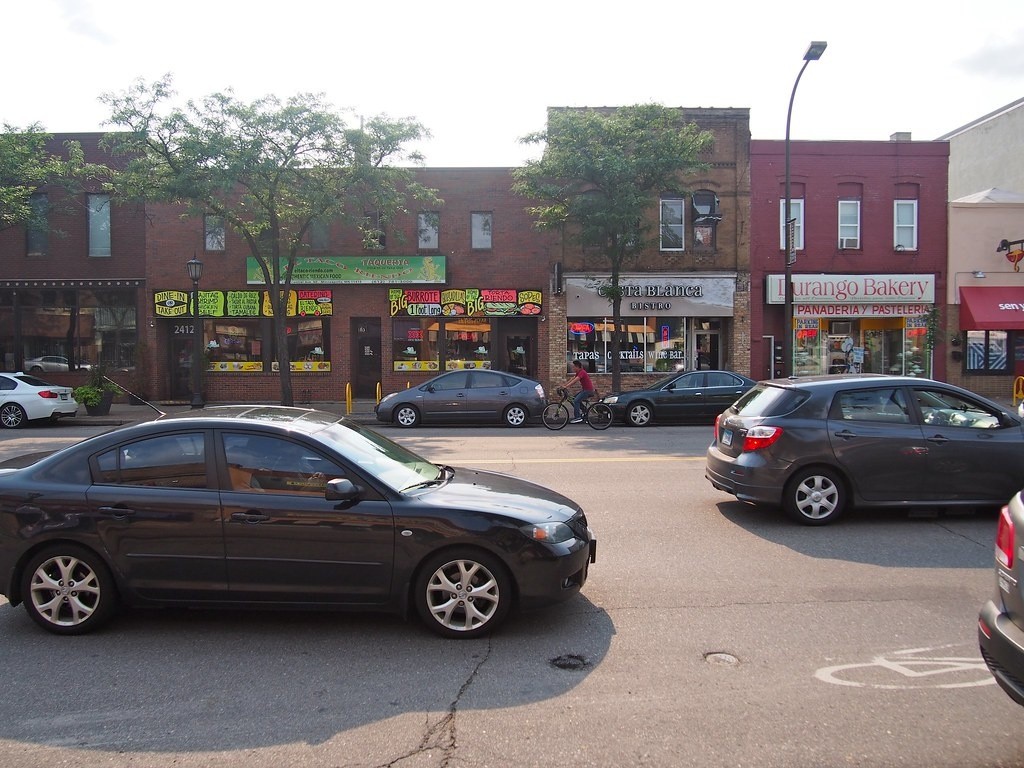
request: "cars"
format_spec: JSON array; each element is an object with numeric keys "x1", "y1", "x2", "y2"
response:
[
  {"x1": 0, "y1": 371, "x2": 79, "y2": 428},
  {"x1": 22, "y1": 356, "x2": 92, "y2": 372},
  {"x1": 374, "y1": 368, "x2": 546, "y2": 428},
  {"x1": 977, "y1": 490, "x2": 1023, "y2": 707},
  {"x1": 704, "y1": 372, "x2": 1024, "y2": 527},
  {"x1": 0, "y1": 404, "x2": 597, "y2": 639},
  {"x1": 599, "y1": 370, "x2": 757, "y2": 427}
]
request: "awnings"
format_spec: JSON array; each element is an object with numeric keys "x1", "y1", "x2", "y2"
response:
[
  {"x1": 567, "y1": 322, "x2": 655, "y2": 333},
  {"x1": 0, "y1": 314, "x2": 95, "y2": 342},
  {"x1": 959, "y1": 285, "x2": 1024, "y2": 331},
  {"x1": 427, "y1": 322, "x2": 490, "y2": 332}
]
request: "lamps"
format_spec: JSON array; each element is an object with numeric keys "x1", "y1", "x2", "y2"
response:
[{"x1": 972, "y1": 271, "x2": 986, "y2": 278}]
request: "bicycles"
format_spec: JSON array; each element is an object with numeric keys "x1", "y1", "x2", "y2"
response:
[{"x1": 542, "y1": 388, "x2": 613, "y2": 431}]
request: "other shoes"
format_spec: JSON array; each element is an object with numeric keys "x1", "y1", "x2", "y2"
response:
[{"x1": 570, "y1": 417, "x2": 582, "y2": 423}]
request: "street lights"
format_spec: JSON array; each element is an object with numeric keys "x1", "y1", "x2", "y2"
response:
[
  {"x1": 784, "y1": 40, "x2": 829, "y2": 374},
  {"x1": 186, "y1": 249, "x2": 205, "y2": 410}
]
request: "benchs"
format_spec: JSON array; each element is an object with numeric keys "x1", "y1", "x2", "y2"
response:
[{"x1": 97, "y1": 445, "x2": 147, "y2": 485}]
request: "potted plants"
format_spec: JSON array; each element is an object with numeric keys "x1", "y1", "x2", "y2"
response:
[{"x1": 73, "y1": 361, "x2": 123, "y2": 416}]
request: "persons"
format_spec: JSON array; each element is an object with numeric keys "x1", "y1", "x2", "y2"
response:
[
  {"x1": 269, "y1": 436, "x2": 327, "y2": 491},
  {"x1": 556, "y1": 360, "x2": 595, "y2": 424}
]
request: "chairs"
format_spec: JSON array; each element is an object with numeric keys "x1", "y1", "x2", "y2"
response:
[
  {"x1": 227, "y1": 445, "x2": 263, "y2": 493},
  {"x1": 246, "y1": 439, "x2": 268, "y2": 469}
]
